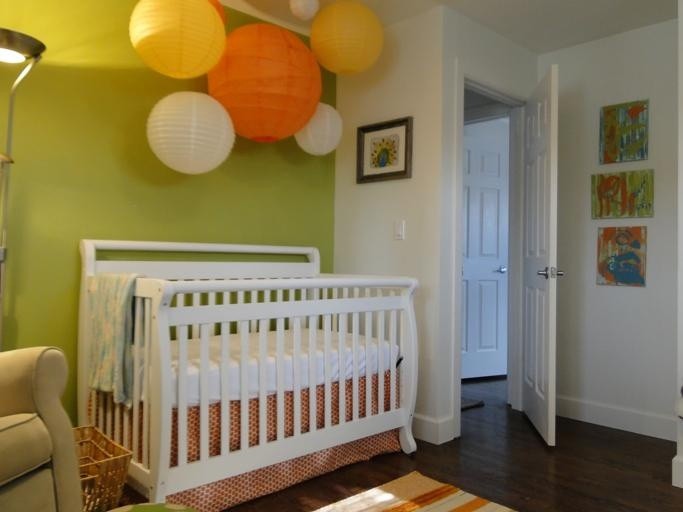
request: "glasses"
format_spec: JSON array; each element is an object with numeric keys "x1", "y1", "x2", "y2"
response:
[
  {"x1": 289, "y1": 0, "x2": 320, "y2": 21},
  {"x1": 294, "y1": 103, "x2": 344, "y2": 156},
  {"x1": 144, "y1": 91, "x2": 236, "y2": 176},
  {"x1": 209, "y1": 1, "x2": 228, "y2": 27},
  {"x1": 310, "y1": 0, "x2": 384, "y2": 76},
  {"x1": 0, "y1": 27, "x2": 47, "y2": 325},
  {"x1": 206, "y1": 22, "x2": 322, "y2": 143},
  {"x1": 128, "y1": 1, "x2": 227, "y2": 79}
]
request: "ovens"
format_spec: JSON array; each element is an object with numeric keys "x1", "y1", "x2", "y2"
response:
[{"x1": 75, "y1": 239, "x2": 418, "y2": 512}]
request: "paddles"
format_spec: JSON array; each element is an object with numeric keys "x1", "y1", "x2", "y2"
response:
[
  {"x1": 312, "y1": 469, "x2": 518, "y2": 512},
  {"x1": 461, "y1": 396, "x2": 485, "y2": 411}
]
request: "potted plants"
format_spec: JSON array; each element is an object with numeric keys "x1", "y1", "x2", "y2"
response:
[{"x1": 355, "y1": 116, "x2": 413, "y2": 184}]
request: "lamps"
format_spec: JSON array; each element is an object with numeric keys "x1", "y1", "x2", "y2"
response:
[{"x1": 0, "y1": 346, "x2": 84, "y2": 512}]
